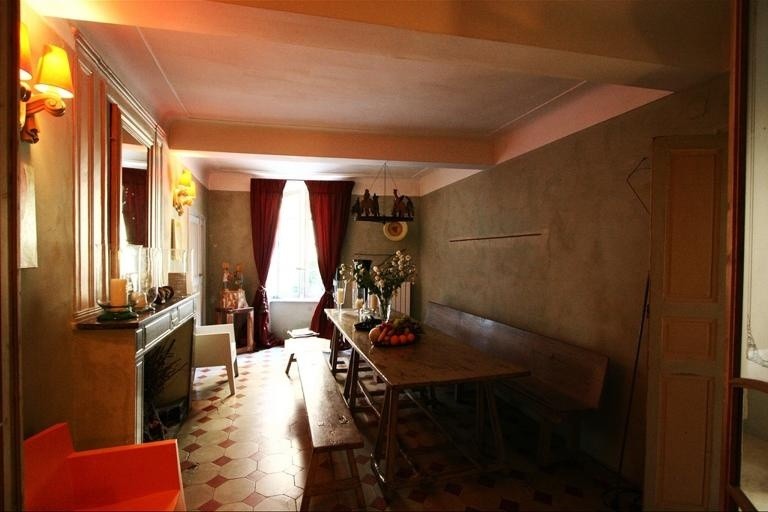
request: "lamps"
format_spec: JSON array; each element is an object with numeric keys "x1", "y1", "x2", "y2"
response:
[
  {"x1": 168, "y1": 160, "x2": 197, "y2": 216},
  {"x1": 18, "y1": 23, "x2": 75, "y2": 144}
]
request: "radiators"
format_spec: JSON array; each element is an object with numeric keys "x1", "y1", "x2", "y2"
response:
[{"x1": 351, "y1": 282, "x2": 411, "y2": 317}]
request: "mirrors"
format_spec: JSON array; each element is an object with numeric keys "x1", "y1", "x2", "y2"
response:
[{"x1": 111, "y1": 109, "x2": 161, "y2": 296}]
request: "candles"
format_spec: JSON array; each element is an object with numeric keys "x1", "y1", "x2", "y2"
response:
[{"x1": 109, "y1": 278, "x2": 129, "y2": 308}]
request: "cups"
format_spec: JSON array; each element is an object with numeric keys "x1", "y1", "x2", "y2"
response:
[{"x1": 120, "y1": 244, "x2": 188, "y2": 312}]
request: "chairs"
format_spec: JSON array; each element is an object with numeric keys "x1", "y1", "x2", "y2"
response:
[
  {"x1": 188, "y1": 318, "x2": 240, "y2": 398},
  {"x1": 20, "y1": 422, "x2": 188, "y2": 512}
]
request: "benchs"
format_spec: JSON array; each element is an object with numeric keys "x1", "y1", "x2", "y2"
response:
[
  {"x1": 284, "y1": 346, "x2": 367, "y2": 511},
  {"x1": 423, "y1": 300, "x2": 610, "y2": 472}
]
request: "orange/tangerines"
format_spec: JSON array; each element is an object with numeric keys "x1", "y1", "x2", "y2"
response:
[
  {"x1": 390, "y1": 335, "x2": 399, "y2": 344},
  {"x1": 407, "y1": 333, "x2": 415, "y2": 341},
  {"x1": 399, "y1": 334, "x2": 406, "y2": 344}
]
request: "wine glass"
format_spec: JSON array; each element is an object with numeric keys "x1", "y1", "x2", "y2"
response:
[{"x1": 333, "y1": 280, "x2": 379, "y2": 318}]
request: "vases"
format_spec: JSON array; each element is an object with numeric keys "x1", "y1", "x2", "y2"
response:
[{"x1": 379, "y1": 301, "x2": 392, "y2": 325}]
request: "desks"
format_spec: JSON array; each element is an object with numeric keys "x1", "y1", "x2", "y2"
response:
[{"x1": 322, "y1": 304, "x2": 532, "y2": 501}]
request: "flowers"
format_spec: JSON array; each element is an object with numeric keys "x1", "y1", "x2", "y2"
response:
[{"x1": 339, "y1": 250, "x2": 418, "y2": 322}]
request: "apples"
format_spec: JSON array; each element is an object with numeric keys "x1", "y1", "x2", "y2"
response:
[{"x1": 369, "y1": 327, "x2": 380, "y2": 342}]
